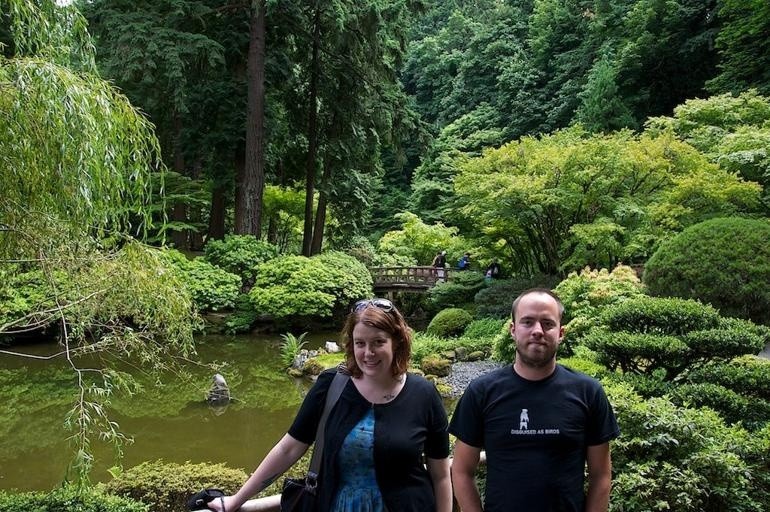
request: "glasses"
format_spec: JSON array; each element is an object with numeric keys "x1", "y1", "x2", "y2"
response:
[{"x1": 352, "y1": 299, "x2": 393, "y2": 312}]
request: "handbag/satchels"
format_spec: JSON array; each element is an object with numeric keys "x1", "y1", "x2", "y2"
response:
[{"x1": 280, "y1": 477, "x2": 317, "y2": 512}]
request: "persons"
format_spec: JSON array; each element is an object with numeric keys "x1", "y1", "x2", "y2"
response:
[
  {"x1": 206, "y1": 296, "x2": 454, "y2": 512},
  {"x1": 448, "y1": 286, "x2": 620, "y2": 512},
  {"x1": 432, "y1": 252, "x2": 446, "y2": 269},
  {"x1": 459, "y1": 252, "x2": 472, "y2": 271},
  {"x1": 487, "y1": 257, "x2": 501, "y2": 280}
]
